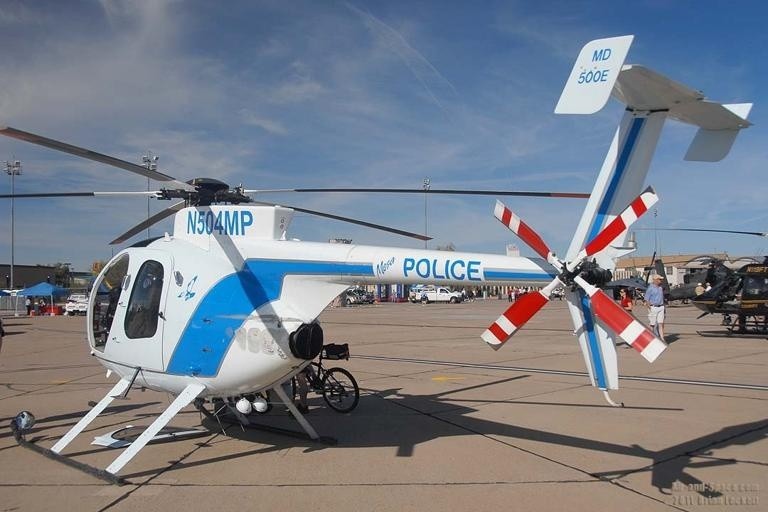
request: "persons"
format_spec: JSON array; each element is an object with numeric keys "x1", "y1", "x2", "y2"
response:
[
  {"x1": 24, "y1": 296, "x2": 47, "y2": 317},
  {"x1": 354, "y1": 285, "x2": 566, "y2": 304},
  {"x1": 288, "y1": 367, "x2": 309, "y2": 417},
  {"x1": 616, "y1": 288, "x2": 635, "y2": 349},
  {"x1": 643, "y1": 273, "x2": 667, "y2": 343},
  {"x1": 632, "y1": 281, "x2": 712, "y2": 305}
]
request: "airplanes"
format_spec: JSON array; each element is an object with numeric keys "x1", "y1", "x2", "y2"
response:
[{"x1": 600, "y1": 259, "x2": 706, "y2": 302}]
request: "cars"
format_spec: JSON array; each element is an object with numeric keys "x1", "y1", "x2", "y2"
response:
[{"x1": 347, "y1": 289, "x2": 375, "y2": 305}]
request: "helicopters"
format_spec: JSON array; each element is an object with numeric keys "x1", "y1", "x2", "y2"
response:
[
  {"x1": 0, "y1": 35, "x2": 753, "y2": 487},
  {"x1": 693, "y1": 256, "x2": 767, "y2": 339}
]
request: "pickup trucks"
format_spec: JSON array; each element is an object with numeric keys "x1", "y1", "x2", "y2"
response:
[
  {"x1": 416, "y1": 288, "x2": 464, "y2": 303},
  {"x1": 66, "y1": 294, "x2": 88, "y2": 315}
]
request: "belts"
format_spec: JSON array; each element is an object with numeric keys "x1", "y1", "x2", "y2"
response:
[{"x1": 649, "y1": 304, "x2": 664, "y2": 307}]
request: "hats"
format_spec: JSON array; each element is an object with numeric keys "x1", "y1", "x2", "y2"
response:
[
  {"x1": 697, "y1": 282, "x2": 703, "y2": 286},
  {"x1": 706, "y1": 283, "x2": 711, "y2": 286},
  {"x1": 652, "y1": 274, "x2": 665, "y2": 281}
]
